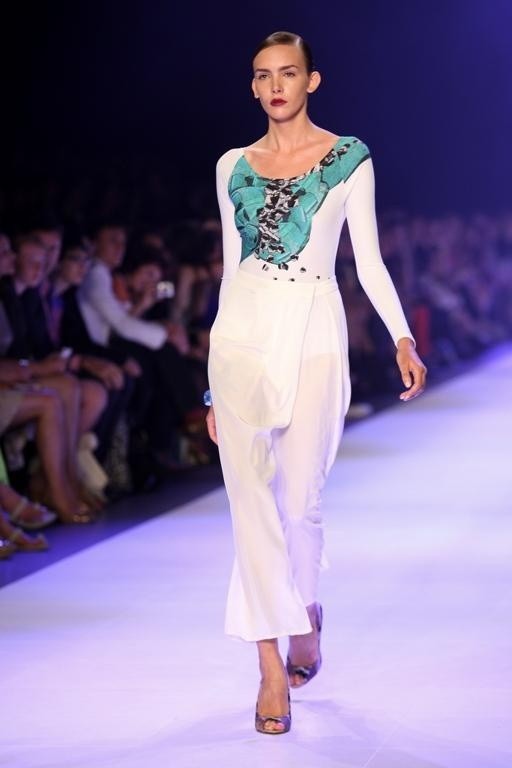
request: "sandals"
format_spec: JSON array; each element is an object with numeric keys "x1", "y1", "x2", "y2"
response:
[
  {"x1": 4, "y1": 531, "x2": 49, "y2": 551},
  {"x1": 6, "y1": 499, "x2": 56, "y2": 528}
]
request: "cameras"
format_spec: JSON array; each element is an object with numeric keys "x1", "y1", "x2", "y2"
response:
[{"x1": 153, "y1": 281, "x2": 175, "y2": 300}]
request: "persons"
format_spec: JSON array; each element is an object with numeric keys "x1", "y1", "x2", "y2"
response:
[
  {"x1": 206, "y1": 30, "x2": 429, "y2": 735},
  {"x1": 335, "y1": 212, "x2": 512, "y2": 425},
  {"x1": 2, "y1": 214, "x2": 225, "y2": 562}
]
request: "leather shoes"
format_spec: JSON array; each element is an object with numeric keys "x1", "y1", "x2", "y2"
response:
[
  {"x1": 255, "y1": 672, "x2": 291, "y2": 734},
  {"x1": 286, "y1": 607, "x2": 323, "y2": 687}
]
